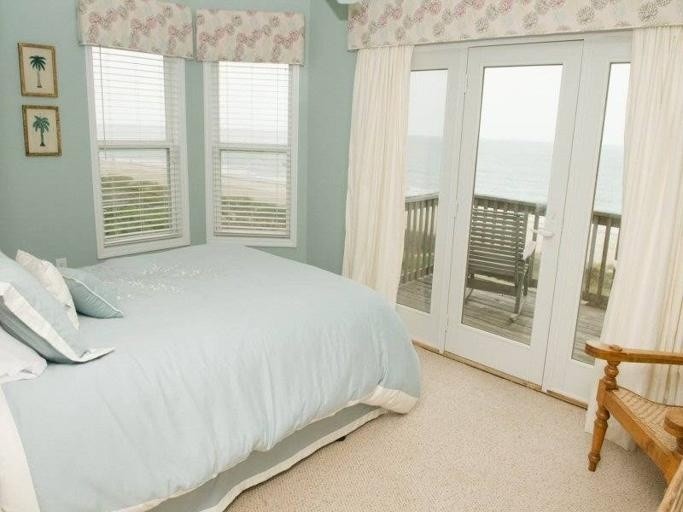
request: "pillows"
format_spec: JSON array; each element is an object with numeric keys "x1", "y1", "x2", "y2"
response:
[
  {"x1": 17, "y1": 249, "x2": 79, "y2": 329},
  {"x1": 56, "y1": 267, "x2": 123, "y2": 318},
  {"x1": 0, "y1": 249, "x2": 114, "y2": 364}
]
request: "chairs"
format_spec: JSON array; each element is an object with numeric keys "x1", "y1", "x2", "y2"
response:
[
  {"x1": 464, "y1": 206, "x2": 536, "y2": 321},
  {"x1": 583, "y1": 339, "x2": 683, "y2": 487}
]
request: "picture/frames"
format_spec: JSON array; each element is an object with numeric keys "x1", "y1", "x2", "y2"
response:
[
  {"x1": 22, "y1": 105, "x2": 63, "y2": 157},
  {"x1": 47, "y1": 75, "x2": 54, "y2": 76},
  {"x1": 18, "y1": 43, "x2": 58, "y2": 97}
]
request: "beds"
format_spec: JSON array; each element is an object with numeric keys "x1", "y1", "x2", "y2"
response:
[{"x1": 0, "y1": 244, "x2": 421, "y2": 512}]
